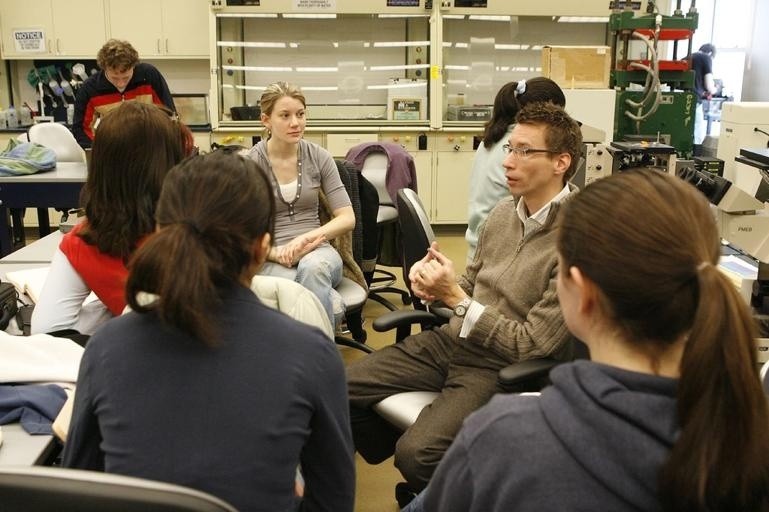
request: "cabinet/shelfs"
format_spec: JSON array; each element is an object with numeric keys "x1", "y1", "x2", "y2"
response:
[
  {"x1": 0, "y1": 1, "x2": 110, "y2": 65},
  {"x1": 205, "y1": 0, "x2": 619, "y2": 228},
  {"x1": 102, "y1": 1, "x2": 212, "y2": 64}
]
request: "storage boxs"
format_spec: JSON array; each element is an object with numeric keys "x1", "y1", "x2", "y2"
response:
[{"x1": 540, "y1": 44, "x2": 613, "y2": 91}]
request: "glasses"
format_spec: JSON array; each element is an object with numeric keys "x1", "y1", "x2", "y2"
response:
[{"x1": 502, "y1": 144, "x2": 563, "y2": 158}]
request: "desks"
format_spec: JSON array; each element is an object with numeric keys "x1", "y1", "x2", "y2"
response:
[
  {"x1": 0, "y1": 161, "x2": 89, "y2": 263},
  {"x1": 0, "y1": 229, "x2": 66, "y2": 263},
  {"x1": 0, "y1": 261, "x2": 103, "y2": 470}
]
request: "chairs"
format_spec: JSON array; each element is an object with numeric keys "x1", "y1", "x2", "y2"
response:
[
  {"x1": 0, "y1": 462, "x2": 239, "y2": 511},
  {"x1": 311, "y1": 136, "x2": 462, "y2": 361},
  {"x1": 365, "y1": 302, "x2": 568, "y2": 511}
]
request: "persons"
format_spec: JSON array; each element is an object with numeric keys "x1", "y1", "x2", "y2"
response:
[
  {"x1": 466, "y1": 77, "x2": 566, "y2": 271},
  {"x1": 73, "y1": 39, "x2": 177, "y2": 150},
  {"x1": 30, "y1": 102, "x2": 185, "y2": 339},
  {"x1": 344, "y1": 102, "x2": 583, "y2": 496},
  {"x1": 680, "y1": 44, "x2": 718, "y2": 144},
  {"x1": 242, "y1": 83, "x2": 357, "y2": 338},
  {"x1": 60, "y1": 149, "x2": 357, "y2": 512},
  {"x1": 398, "y1": 168, "x2": 769, "y2": 512}
]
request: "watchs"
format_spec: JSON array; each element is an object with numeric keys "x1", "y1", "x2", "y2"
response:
[{"x1": 453, "y1": 298, "x2": 471, "y2": 317}]
